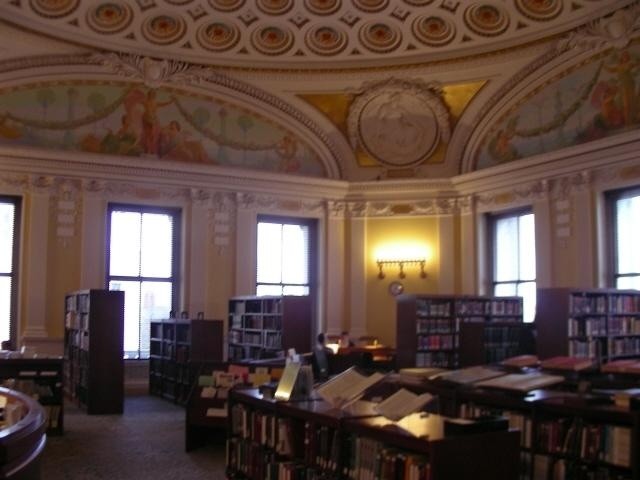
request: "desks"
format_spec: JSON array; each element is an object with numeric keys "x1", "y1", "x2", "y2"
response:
[{"x1": 1, "y1": 339, "x2": 67, "y2": 437}]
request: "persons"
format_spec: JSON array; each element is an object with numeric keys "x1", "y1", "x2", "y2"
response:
[
  {"x1": 337, "y1": 332, "x2": 354, "y2": 348},
  {"x1": 318, "y1": 333, "x2": 333, "y2": 359}
]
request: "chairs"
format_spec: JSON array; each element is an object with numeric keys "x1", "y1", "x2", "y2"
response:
[{"x1": 313, "y1": 347, "x2": 378, "y2": 382}]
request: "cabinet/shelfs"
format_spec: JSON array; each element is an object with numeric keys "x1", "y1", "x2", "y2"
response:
[
  {"x1": 368, "y1": 354, "x2": 640, "y2": 479},
  {"x1": 184, "y1": 359, "x2": 313, "y2": 449},
  {"x1": 393, "y1": 283, "x2": 640, "y2": 368},
  {"x1": 227, "y1": 383, "x2": 522, "y2": 478},
  {"x1": 61, "y1": 287, "x2": 313, "y2": 416}
]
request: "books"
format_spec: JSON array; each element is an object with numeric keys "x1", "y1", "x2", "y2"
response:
[
  {"x1": 228, "y1": 300, "x2": 282, "y2": 362},
  {"x1": 61, "y1": 295, "x2": 90, "y2": 402},
  {"x1": 415, "y1": 298, "x2": 522, "y2": 368},
  {"x1": 2, "y1": 347, "x2": 64, "y2": 429},
  {"x1": 199, "y1": 350, "x2": 640, "y2": 480},
  {"x1": 567, "y1": 296, "x2": 639, "y2": 361}
]
